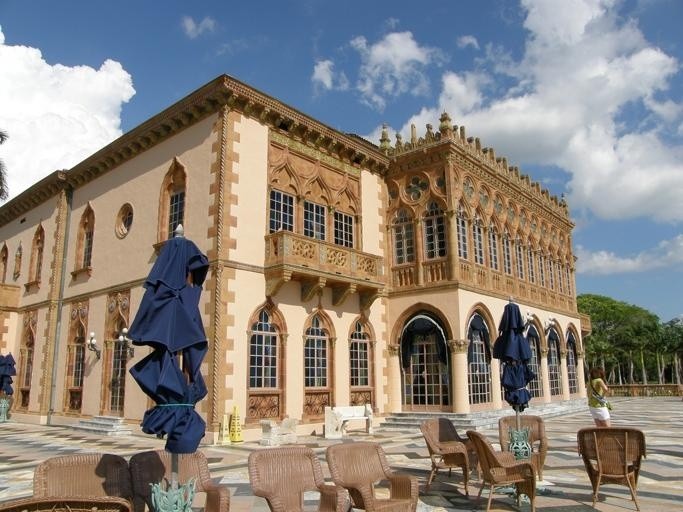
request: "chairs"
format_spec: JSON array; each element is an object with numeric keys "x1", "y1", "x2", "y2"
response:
[
  {"x1": 34, "y1": 453, "x2": 134, "y2": 512},
  {"x1": 577, "y1": 427, "x2": 646, "y2": 512},
  {"x1": 420, "y1": 418, "x2": 481, "y2": 497},
  {"x1": 499, "y1": 416, "x2": 547, "y2": 482},
  {"x1": 0, "y1": 496, "x2": 134, "y2": 512},
  {"x1": 249, "y1": 445, "x2": 345, "y2": 512},
  {"x1": 327, "y1": 443, "x2": 419, "y2": 512},
  {"x1": 466, "y1": 431, "x2": 536, "y2": 512},
  {"x1": 130, "y1": 449, "x2": 230, "y2": 512}
]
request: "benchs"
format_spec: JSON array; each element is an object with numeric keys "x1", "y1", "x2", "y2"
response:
[{"x1": 324, "y1": 404, "x2": 373, "y2": 439}]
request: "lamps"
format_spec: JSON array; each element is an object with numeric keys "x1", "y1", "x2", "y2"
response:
[
  {"x1": 86, "y1": 331, "x2": 100, "y2": 359},
  {"x1": 118, "y1": 328, "x2": 134, "y2": 357}
]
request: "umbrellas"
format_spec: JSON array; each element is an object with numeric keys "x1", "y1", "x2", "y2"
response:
[
  {"x1": 493, "y1": 295, "x2": 535, "y2": 430},
  {"x1": 125, "y1": 223, "x2": 210, "y2": 488},
  {"x1": 0, "y1": 352, "x2": 16, "y2": 399}
]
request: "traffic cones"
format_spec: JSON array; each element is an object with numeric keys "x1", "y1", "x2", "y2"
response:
[{"x1": 230, "y1": 405, "x2": 243, "y2": 442}]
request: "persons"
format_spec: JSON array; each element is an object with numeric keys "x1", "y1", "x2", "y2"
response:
[{"x1": 587, "y1": 366, "x2": 612, "y2": 427}]
